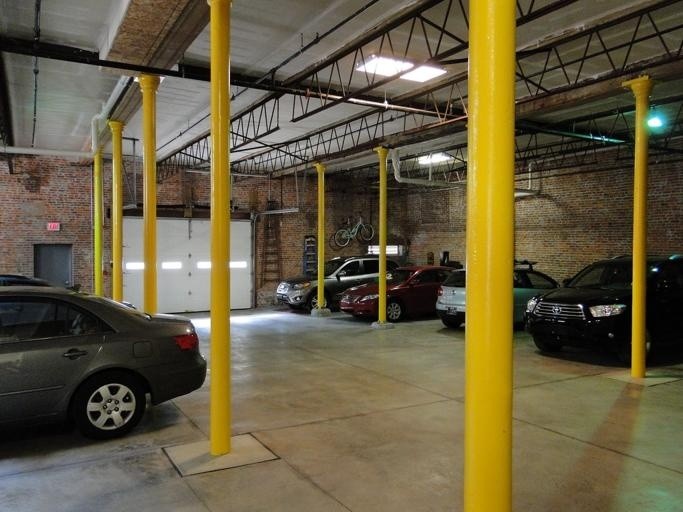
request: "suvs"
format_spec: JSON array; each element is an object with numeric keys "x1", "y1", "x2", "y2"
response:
[
  {"x1": 275, "y1": 253, "x2": 401, "y2": 312},
  {"x1": 524, "y1": 254, "x2": 683, "y2": 361}
]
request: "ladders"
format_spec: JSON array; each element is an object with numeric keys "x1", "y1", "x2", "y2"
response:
[
  {"x1": 304, "y1": 235, "x2": 318, "y2": 274},
  {"x1": 260, "y1": 201, "x2": 282, "y2": 288}
]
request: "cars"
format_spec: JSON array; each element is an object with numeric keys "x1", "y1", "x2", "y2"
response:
[
  {"x1": 0, "y1": 284, "x2": 206, "y2": 440},
  {"x1": 436, "y1": 263, "x2": 560, "y2": 327},
  {"x1": 338, "y1": 262, "x2": 454, "y2": 322}
]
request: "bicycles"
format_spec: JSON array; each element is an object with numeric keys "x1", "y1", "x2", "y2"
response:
[{"x1": 333, "y1": 214, "x2": 376, "y2": 248}]
